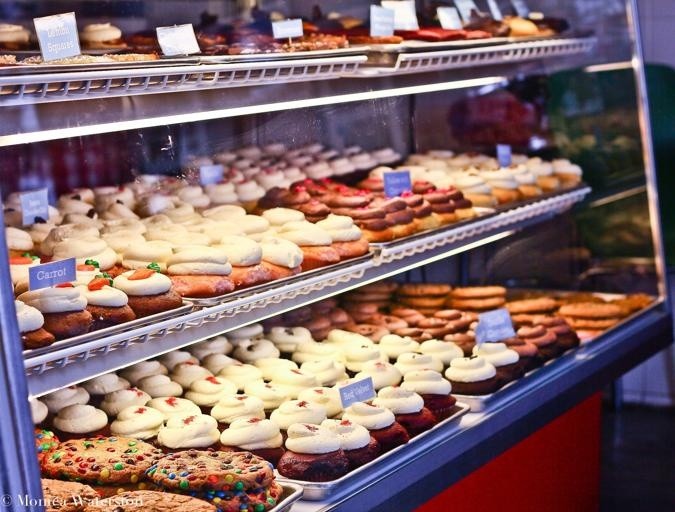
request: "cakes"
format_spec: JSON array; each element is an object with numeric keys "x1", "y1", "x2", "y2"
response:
[{"x1": 0, "y1": 11, "x2": 659, "y2": 512}]
